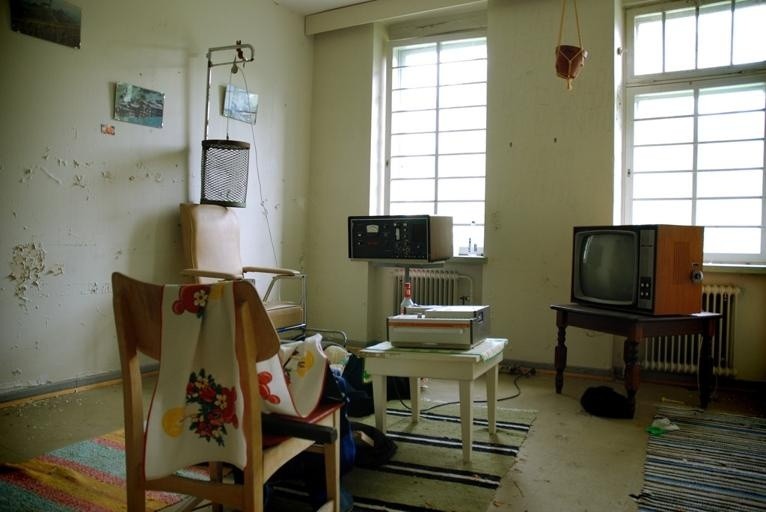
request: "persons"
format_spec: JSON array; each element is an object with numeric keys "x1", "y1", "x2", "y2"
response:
[{"x1": 118, "y1": 87, "x2": 163, "y2": 111}]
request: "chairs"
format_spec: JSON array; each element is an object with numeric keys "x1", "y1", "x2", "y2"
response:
[
  {"x1": 176, "y1": 201, "x2": 307, "y2": 336},
  {"x1": 110, "y1": 269, "x2": 341, "y2": 512}
]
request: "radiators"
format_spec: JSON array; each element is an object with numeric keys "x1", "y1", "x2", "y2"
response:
[
  {"x1": 392, "y1": 269, "x2": 474, "y2": 317},
  {"x1": 638, "y1": 285, "x2": 743, "y2": 377}
]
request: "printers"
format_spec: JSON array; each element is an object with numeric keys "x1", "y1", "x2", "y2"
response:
[{"x1": 388, "y1": 304, "x2": 490, "y2": 349}]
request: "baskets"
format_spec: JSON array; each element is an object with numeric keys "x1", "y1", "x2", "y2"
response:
[{"x1": 198, "y1": 139, "x2": 251, "y2": 208}]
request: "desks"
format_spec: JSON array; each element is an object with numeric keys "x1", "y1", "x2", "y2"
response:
[
  {"x1": 357, "y1": 336, "x2": 510, "y2": 465},
  {"x1": 550, "y1": 304, "x2": 724, "y2": 418}
]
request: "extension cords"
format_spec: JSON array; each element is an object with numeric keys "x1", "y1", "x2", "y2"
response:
[{"x1": 499, "y1": 364, "x2": 536, "y2": 375}]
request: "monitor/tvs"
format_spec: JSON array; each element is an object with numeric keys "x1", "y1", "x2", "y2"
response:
[{"x1": 571, "y1": 226, "x2": 703, "y2": 316}]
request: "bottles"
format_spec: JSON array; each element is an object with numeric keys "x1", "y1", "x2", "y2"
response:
[{"x1": 400, "y1": 283, "x2": 414, "y2": 314}]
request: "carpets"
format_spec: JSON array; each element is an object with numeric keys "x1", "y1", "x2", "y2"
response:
[{"x1": 636, "y1": 397, "x2": 766, "y2": 511}]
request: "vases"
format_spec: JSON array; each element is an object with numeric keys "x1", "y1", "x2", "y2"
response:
[{"x1": 556, "y1": 44, "x2": 588, "y2": 81}]
